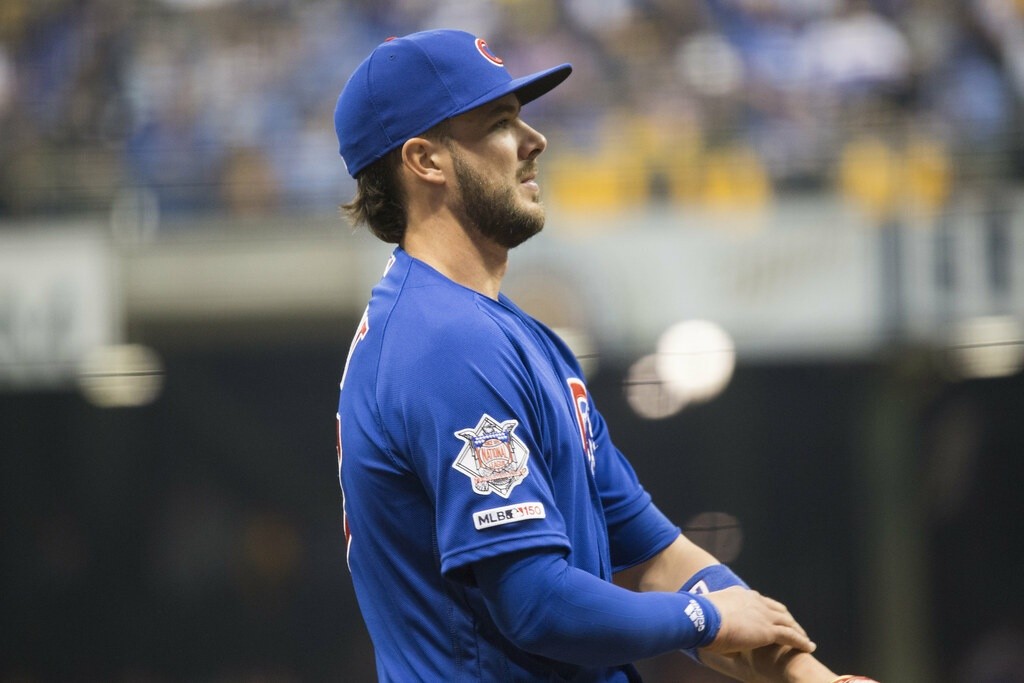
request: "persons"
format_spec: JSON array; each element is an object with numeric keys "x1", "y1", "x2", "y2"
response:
[{"x1": 327, "y1": 26, "x2": 851, "y2": 682}]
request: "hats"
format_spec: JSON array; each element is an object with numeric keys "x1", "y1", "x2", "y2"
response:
[{"x1": 334, "y1": 29, "x2": 572, "y2": 179}]
